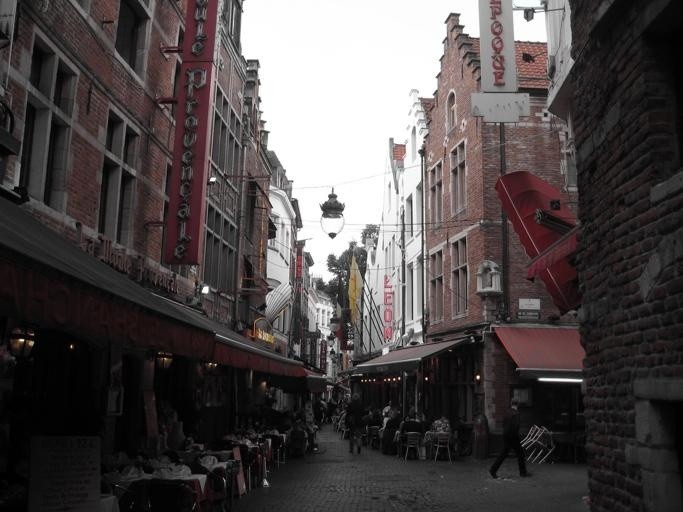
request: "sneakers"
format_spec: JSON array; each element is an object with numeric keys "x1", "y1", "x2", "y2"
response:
[{"x1": 488, "y1": 468, "x2": 532, "y2": 480}]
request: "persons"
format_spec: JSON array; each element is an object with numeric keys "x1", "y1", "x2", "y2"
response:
[
  {"x1": 341, "y1": 391, "x2": 452, "y2": 460},
  {"x1": 158, "y1": 398, "x2": 337, "y2": 490},
  {"x1": 488, "y1": 396, "x2": 532, "y2": 479}
]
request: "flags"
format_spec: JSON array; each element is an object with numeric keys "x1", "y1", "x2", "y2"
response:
[{"x1": 347, "y1": 255, "x2": 364, "y2": 325}]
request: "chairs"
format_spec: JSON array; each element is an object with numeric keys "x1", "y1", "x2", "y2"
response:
[
  {"x1": 331, "y1": 408, "x2": 452, "y2": 465},
  {"x1": 520, "y1": 423, "x2": 556, "y2": 465},
  {"x1": 102, "y1": 427, "x2": 289, "y2": 512}
]
